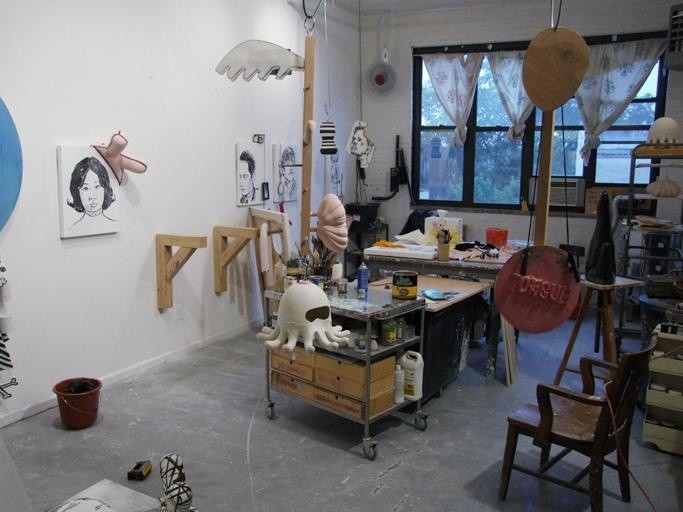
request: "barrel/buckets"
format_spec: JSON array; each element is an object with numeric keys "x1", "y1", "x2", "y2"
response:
[
  {"x1": 345, "y1": 202, "x2": 378, "y2": 232},
  {"x1": 396, "y1": 350, "x2": 424, "y2": 402}
]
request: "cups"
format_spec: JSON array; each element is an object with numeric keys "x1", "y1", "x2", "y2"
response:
[
  {"x1": 284, "y1": 259, "x2": 332, "y2": 291},
  {"x1": 337, "y1": 277, "x2": 348, "y2": 295},
  {"x1": 437, "y1": 243, "x2": 450, "y2": 262}
]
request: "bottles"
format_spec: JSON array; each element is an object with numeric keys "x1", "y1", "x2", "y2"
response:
[
  {"x1": 379, "y1": 318, "x2": 414, "y2": 346},
  {"x1": 357, "y1": 262, "x2": 369, "y2": 301}
]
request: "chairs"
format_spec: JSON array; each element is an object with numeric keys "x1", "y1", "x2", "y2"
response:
[{"x1": 498, "y1": 332, "x2": 659, "y2": 511}]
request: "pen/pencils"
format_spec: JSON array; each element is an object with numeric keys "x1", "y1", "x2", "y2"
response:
[
  {"x1": 450, "y1": 275, "x2": 481, "y2": 282},
  {"x1": 437, "y1": 228, "x2": 452, "y2": 244}
]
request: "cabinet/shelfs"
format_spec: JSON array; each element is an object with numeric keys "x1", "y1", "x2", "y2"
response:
[{"x1": 616, "y1": 142, "x2": 683, "y2": 456}]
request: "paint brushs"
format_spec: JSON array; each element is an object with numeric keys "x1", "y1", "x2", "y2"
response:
[{"x1": 295, "y1": 240, "x2": 338, "y2": 272}]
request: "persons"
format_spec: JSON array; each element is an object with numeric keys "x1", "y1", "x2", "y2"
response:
[
  {"x1": 237, "y1": 151, "x2": 260, "y2": 206},
  {"x1": 277, "y1": 147, "x2": 297, "y2": 203},
  {"x1": 349, "y1": 126, "x2": 375, "y2": 170},
  {"x1": 61, "y1": 157, "x2": 117, "y2": 238}
]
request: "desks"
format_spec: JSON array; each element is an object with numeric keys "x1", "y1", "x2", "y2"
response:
[
  {"x1": 343, "y1": 224, "x2": 388, "y2": 280},
  {"x1": 554, "y1": 273, "x2": 646, "y2": 386},
  {"x1": 370, "y1": 274, "x2": 492, "y2": 407},
  {"x1": 368, "y1": 255, "x2": 504, "y2": 385}
]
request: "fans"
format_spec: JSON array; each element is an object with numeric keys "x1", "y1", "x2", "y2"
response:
[{"x1": 366, "y1": 61, "x2": 398, "y2": 96}]
